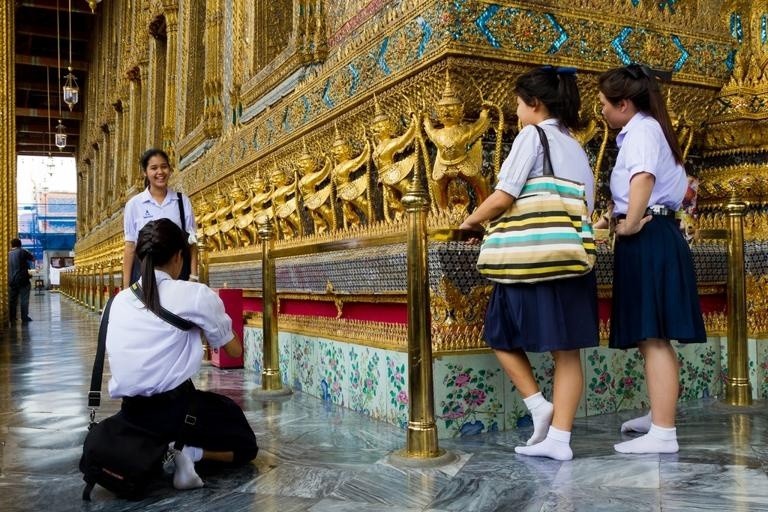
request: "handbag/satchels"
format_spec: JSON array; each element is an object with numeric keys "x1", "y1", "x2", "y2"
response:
[
  {"x1": 79, "y1": 409, "x2": 173, "y2": 502},
  {"x1": 476, "y1": 176, "x2": 597, "y2": 287},
  {"x1": 182, "y1": 233, "x2": 193, "y2": 283}
]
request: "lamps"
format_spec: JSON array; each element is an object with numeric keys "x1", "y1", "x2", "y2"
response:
[{"x1": 46, "y1": 0, "x2": 101, "y2": 176}]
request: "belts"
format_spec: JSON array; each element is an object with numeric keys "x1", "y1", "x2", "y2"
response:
[{"x1": 617, "y1": 206, "x2": 677, "y2": 218}]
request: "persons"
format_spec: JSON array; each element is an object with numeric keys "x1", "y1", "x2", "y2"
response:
[
  {"x1": 8, "y1": 320, "x2": 34, "y2": 399},
  {"x1": 201, "y1": 160, "x2": 276, "y2": 253},
  {"x1": 371, "y1": 93, "x2": 416, "y2": 224},
  {"x1": 454, "y1": 65, "x2": 599, "y2": 463},
  {"x1": 271, "y1": 137, "x2": 333, "y2": 235},
  {"x1": 424, "y1": 97, "x2": 490, "y2": 217},
  {"x1": 592, "y1": 64, "x2": 707, "y2": 455},
  {"x1": 331, "y1": 120, "x2": 375, "y2": 225},
  {"x1": 99, "y1": 217, "x2": 260, "y2": 491},
  {"x1": 7, "y1": 237, "x2": 38, "y2": 323},
  {"x1": 119, "y1": 147, "x2": 200, "y2": 290}
]
